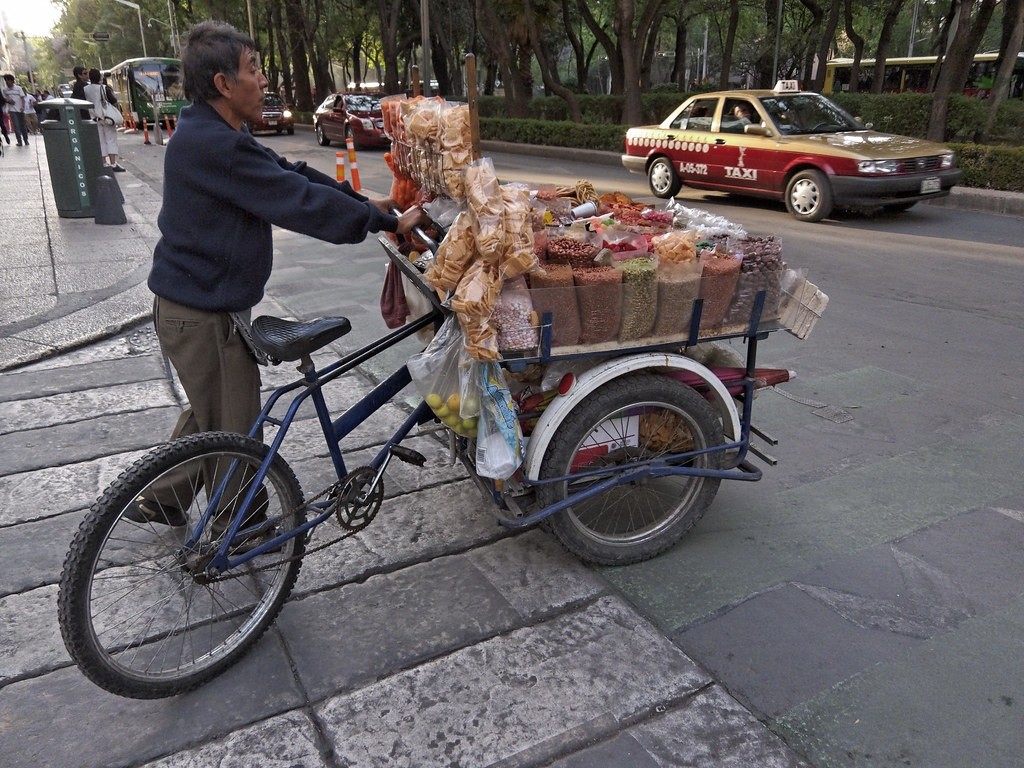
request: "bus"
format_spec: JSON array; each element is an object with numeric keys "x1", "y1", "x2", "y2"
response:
[
  {"x1": 105, "y1": 57, "x2": 192, "y2": 130},
  {"x1": 822, "y1": 52, "x2": 1024, "y2": 102}
]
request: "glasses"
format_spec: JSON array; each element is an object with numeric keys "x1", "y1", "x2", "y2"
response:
[{"x1": 79, "y1": 73, "x2": 88, "y2": 75}]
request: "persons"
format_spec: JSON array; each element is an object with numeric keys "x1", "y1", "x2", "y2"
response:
[
  {"x1": 0, "y1": 89, "x2": 15, "y2": 155},
  {"x1": 22, "y1": 87, "x2": 54, "y2": 135},
  {"x1": 1, "y1": 74, "x2": 30, "y2": 146},
  {"x1": 71, "y1": 67, "x2": 91, "y2": 120},
  {"x1": 776, "y1": 103, "x2": 800, "y2": 130},
  {"x1": 84, "y1": 69, "x2": 126, "y2": 172},
  {"x1": 729, "y1": 103, "x2": 754, "y2": 123},
  {"x1": 123, "y1": 18, "x2": 433, "y2": 553},
  {"x1": 171, "y1": 77, "x2": 182, "y2": 87}
]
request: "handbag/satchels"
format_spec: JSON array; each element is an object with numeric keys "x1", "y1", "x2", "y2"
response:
[{"x1": 99, "y1": 83, "x2": 125, "y2": 126}]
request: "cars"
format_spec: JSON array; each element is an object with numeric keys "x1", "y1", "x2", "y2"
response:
[
  {"x1": 621, "y1": 79, "x2": 963, "y2": 222},
  {"x1": 58, "y1": 84, "x2": 74, "y2": 98},
  {"x1": 311, "y1": 93, "x2": 392, "y2": 151},
  {"x1": 242, "y1": 91, "x2": 295, "y2": 136}
]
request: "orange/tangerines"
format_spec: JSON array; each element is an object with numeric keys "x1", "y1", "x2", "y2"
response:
[{"x1": 426, "y1": 394, "x2": 478, "y2": 437}]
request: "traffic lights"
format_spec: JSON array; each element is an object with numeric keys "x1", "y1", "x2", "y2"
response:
[{"x1": 32, "y1": 71, "x2": 38, "y2": 80}]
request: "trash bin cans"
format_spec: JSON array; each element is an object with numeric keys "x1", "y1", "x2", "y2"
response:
[{"x1": 37, "y1": 98, "x2": 105, "y2": 219}]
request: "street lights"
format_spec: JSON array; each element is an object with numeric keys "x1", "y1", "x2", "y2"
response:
[{"x1": 148, "y1": 18, "x2": 182, "y2": 56}]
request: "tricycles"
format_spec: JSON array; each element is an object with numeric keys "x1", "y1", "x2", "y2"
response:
[{"x1": 55, "y1": 203, "x2": 794, "y2": 701}]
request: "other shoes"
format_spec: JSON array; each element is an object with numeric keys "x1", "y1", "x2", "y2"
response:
[
  {"x1": 110, "y1": 163, "x2": 126, "y2": 172},
  {"x1": 25, "y1": 141, "x2": 29, "y2": 145},
  {"x1": 211, "y1": 526, "x2": 288, "y2": 555},
  {"x1": 17, "y1": 142, "x2": 22, "y2": 146},
  {"x1": 123, "y1": 492, "x2": 190, "y2": 526},
  {"x1": 6, "y1": 136, "x2": 9, "y2": 144}
]
